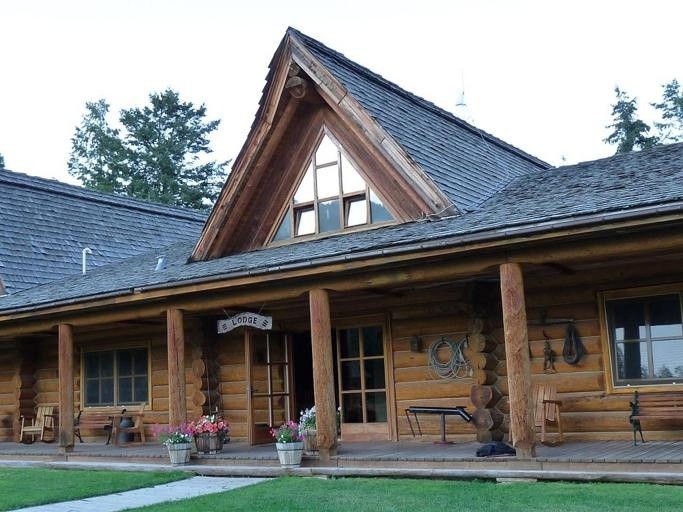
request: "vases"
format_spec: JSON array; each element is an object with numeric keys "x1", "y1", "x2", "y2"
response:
[
  {"x1": 303, "y1": 434, "x2": 319, "y2": 456},
  {"x1": 276, "y1": 443, "x2": 303, "y2": 467},
  {"x1": 193, "y1": 432, "x2": 223, "y2": 454},
  {"x1": 167, "y1": 444, "x2": 192, "y2": 466}
]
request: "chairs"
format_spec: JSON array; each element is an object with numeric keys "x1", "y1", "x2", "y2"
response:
[
  {"x1": 507, "y1": 383, "x2": 564, "y2": 443},
  {"x1": 107, "y1": 399, "x2": 147, "y2": 447},
  {"x1": 19, "y1": 406, "x2": 57, "y2": 446}
]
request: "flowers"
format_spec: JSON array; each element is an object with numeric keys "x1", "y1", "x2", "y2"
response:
[
  {"x1": 149, "y1": 413, "x2": 229, "y2": 446},
  {"x1": 296, "y1": 404, "x2": 341, "y2": 437},
  {"x1": 268, "y1": 421, "x2": 303, "y2": 443}
]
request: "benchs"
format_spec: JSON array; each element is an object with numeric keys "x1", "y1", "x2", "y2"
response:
[
  {"x1": 71, "y1": 409, "x2": 127, "y2": 445},
  {"x1": 629, "y1": 390, "x2": 682, "y2": 445}
]
